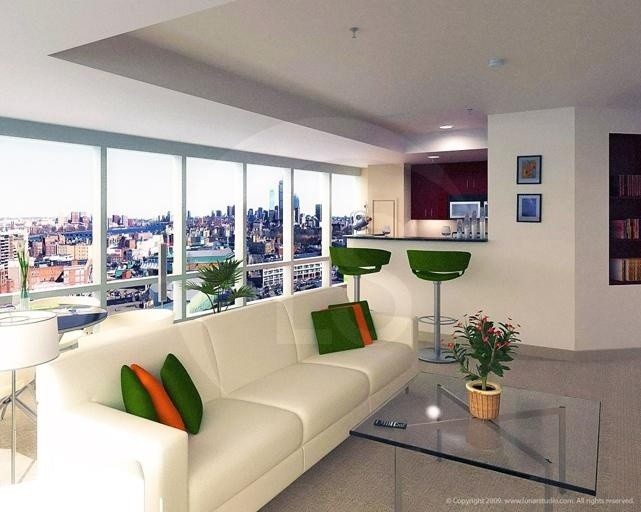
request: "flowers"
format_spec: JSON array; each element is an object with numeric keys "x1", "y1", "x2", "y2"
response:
[
  {"x1": 11, "y1": 240, "x2": 29, "y2": 292},
  {"x1": 443, "y1": 309, "x2": 523, "y2": 389}
]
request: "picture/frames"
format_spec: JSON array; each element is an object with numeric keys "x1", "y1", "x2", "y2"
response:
[
  {"x1": 517, "y1": 193, "x2": 541, "y2": 222},
  {"x1": 517, "y1": 155, "x2": 542, "y2": 184}
]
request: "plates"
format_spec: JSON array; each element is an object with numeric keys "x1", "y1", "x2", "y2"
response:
[{"x1": 371, "y1": 232, "x2": 384, "y2": 236}]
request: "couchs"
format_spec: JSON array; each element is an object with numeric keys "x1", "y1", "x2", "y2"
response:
[{"x1": 35, "y1": 287, "x2": 416, "y2": 512}]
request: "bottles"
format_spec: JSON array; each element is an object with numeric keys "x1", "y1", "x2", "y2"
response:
[
  {"x1": 352, "y1": 217, "x2": 372, "y2": 230},
  {"x1": 480, "y1": 216, "x2": 485, "y2": 239},
  {"x1": 456, "y1": 221, "x2": 463, "y2": 240},
  {"x1": 464, "y1": 214, "x2": 470, "y2": 239},
  {"x1": 472, "y1": 210, "x2": 477, "y2": 239}
]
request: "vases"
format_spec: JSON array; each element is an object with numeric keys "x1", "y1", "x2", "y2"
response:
[
  {"x1": 20, "y1": 284, "x2": 31, "y2": 309},
  {"x1": 466, "y1": 381, "x2": 501, "y2": 420}
]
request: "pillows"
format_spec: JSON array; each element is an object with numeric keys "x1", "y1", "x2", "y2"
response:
[
  {"x1": 161, "y1": 354, "x2": 203, "y2": 434},
  {"x1": 131, "y1": 364, "x2": 184, "y2": 434},
  {"x1": 330, "y1": 300, "x2": 375, "y2": 343},
  {"x1": 121, "y1": 365, "x2": 158, "y2": 422},
  {"x1": 342, "y1": 306, "x2": 372, "y2": 346},
  {"x1": 309, "y1": 308, "x2": 364, "y2": 354}
]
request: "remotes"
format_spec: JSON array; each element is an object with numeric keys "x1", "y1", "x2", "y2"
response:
[{"x1": 374, "y1": 419, "x2": 407, "y2": 429}]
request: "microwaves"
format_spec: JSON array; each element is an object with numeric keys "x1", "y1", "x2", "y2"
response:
[{"x1": 449, "y1": 201, "x2": 481, "y2": 218}]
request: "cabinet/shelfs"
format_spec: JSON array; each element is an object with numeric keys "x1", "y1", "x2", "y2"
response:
[{"x1": 609, "y1": 133, "x2": 641, "y2": 285}]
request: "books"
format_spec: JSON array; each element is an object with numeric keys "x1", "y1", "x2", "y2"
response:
[{"x1": 611, "y1": 174, "x2": 641, "y2": 282}]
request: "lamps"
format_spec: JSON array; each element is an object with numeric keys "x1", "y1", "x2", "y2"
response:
[{"x1": 0, "y1": 308, "x2": 57, "y2": 482}]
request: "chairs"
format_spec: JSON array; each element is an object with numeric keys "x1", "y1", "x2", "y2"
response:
[
  {"x1": 406, "y1": 249, "x2": 472, "y2": 362},
  {"x1": 328, "y1": 246, "x2": 392, "y2": 303}
]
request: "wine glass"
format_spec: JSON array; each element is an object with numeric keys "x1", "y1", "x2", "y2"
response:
[
  {"x1": 382, "y1": 225, "x2": 391, "y2": 237},
  {"x1": 441, "y1": 226, "x2": 451, "y2": 239}
]
